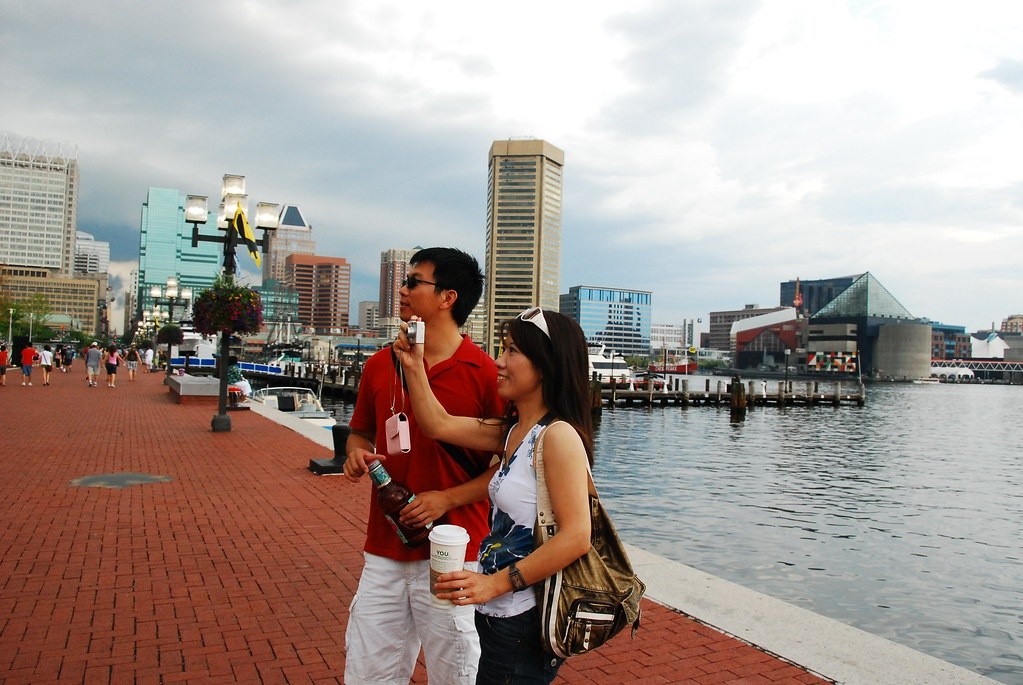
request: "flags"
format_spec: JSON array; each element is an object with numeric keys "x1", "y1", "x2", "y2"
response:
[{"x1": 233, "y1": 202, "x2": 262, "y2": 267}]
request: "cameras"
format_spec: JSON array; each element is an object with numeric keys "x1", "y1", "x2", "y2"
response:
[{"x1": 405, "y1": 321, "x2": 427, "y2": 345}]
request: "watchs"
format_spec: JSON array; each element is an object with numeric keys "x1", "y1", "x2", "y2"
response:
[{"x1": 507, "y1": 562, "x2": 528, "y2": 592}]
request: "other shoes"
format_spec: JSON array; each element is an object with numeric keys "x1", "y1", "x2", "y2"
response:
[
  {"x1": 28, "y1": 383, "x2": 33, "y2": 387},
  {"x1": 22, "y1": 382, "x2": 25, "y2": 386},
  {"x1": 88, "y1": 381, "x2": 92, "y2": 386},
  {"x1": 47, "y1": 380, "x2": 49, "y2": 384},
  {"x1": 94, "y1": 382, "x2": 97, "y2": 387},
  {"x1": 110, "y1": 384, "x2": 116, "y2": 388}
]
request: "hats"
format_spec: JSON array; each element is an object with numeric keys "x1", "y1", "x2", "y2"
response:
[{"x1": 92, "y1": 342, "x2": 98, "y2": 346}]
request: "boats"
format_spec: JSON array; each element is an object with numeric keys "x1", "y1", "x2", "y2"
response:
[
  {"x1": 255, "y1": 313, "x2": 303, "y2": 363},
  {"x1": 250, "y1": 384, "x2": 338, "y2": 431},
  {"x1": 587, "y1": 343, "x2": 632, "y2": 382}
]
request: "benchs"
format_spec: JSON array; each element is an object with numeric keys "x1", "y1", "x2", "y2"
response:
[{"x1": 226, "y1": 382, "x2": 251, "y2": 410}]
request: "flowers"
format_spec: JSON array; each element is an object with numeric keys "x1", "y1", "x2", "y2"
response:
[
  {"x1": 140, "y1": 339, "x2": 156, "y2": 349},
  {"x1": 156, "y1": 324, "x2": 184, "y2": 346},
  {"x1": 191, "y1": 272, "x2": 266, "y2": 337}
]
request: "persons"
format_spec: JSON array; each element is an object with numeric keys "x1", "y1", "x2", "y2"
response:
[
  {"x1": 0, "y1": 334, "x2": 155, "y2": 387},
  {"x1": 344, "y1": 247, "x2": 594, "y2": 685}
]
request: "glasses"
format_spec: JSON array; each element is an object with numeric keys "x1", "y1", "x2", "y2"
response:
[
  {"x1": 515, "y1": 306, "x2": 551, "y2": 339},
  {"x1": 400, "y1": 275, "x2": 445, "y2": 290}
]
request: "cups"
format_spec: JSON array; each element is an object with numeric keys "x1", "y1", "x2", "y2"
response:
[{"x1": 428, "y1": 524, "x2": 471, "y2": 609}]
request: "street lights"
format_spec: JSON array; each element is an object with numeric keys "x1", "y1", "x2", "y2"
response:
[
  {"x1": 28, "y1": 312, "x2": 34, "y2": 342},
  {"x1": 784, "y1": 346, "x2": 792, "y2": 394},
  {"x1": 183, "y1": 173, "x2": 281, "y2": 432},
  {"x1": 8, "y1": 309, "x2": 14, "y2": 345},
  {"x1": 150, "y1": 276, "x2": 191, "y2": 384}
]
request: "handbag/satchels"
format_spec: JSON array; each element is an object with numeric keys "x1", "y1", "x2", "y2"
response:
[
  {"x1": 46, "y1": 364, "x2": 53, "y2": 373},
  {"x1": 535, "y1": 420, "x2": 645, "y2": 659}
]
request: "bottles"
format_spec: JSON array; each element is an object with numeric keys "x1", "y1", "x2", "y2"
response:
[{"x1": 366, "y1": 459, "x2": 434, "y2": 549}]
